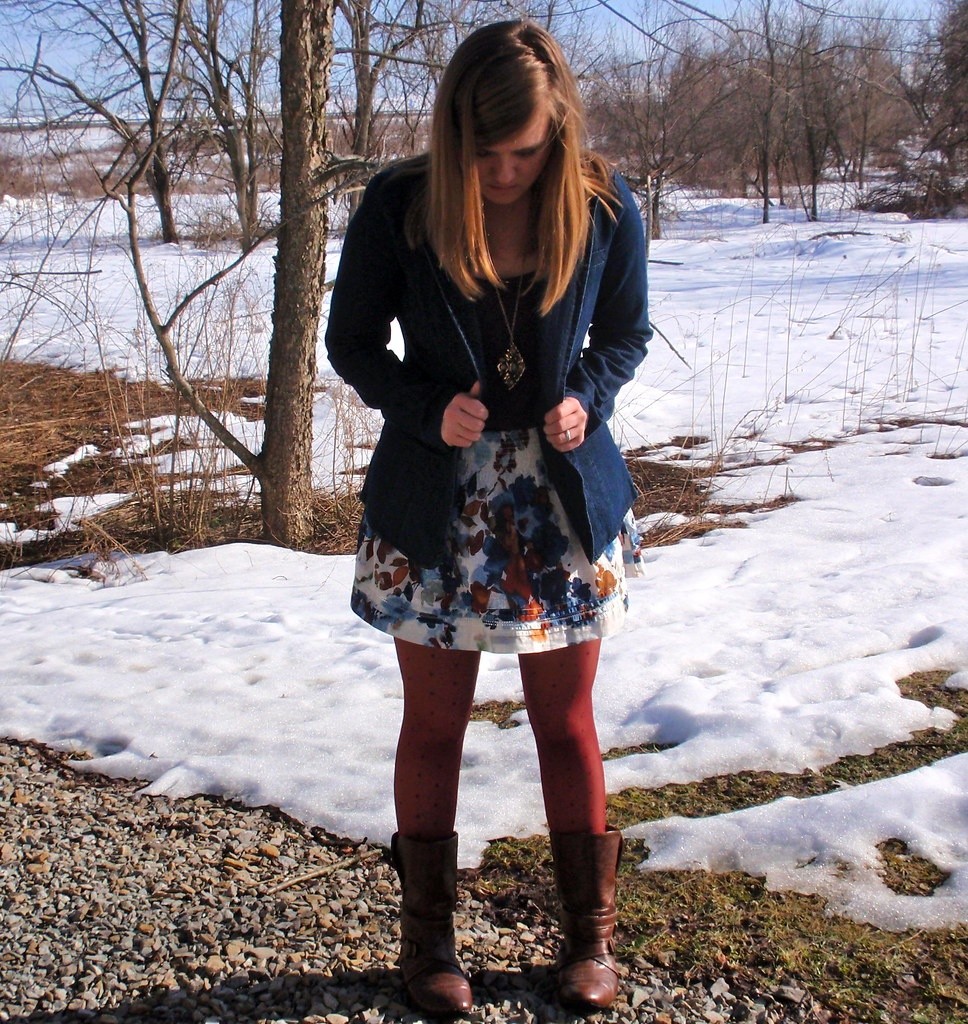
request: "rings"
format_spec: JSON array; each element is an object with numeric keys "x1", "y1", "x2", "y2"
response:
[{"x1": 565, "y1": 429, "x2": 571, "y2": 442}]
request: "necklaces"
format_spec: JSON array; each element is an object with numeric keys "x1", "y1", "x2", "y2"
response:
[{"x1": 482, "y1": 196, "x2": 527, "y2": 392}]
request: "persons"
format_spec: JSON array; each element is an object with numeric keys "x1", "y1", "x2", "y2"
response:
[{"x1": 323, "y1": 15, "x2": 657, "y2": 1019}]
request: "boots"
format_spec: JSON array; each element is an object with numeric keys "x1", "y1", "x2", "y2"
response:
[
  {"x1": 391, "y1": 833, "x2": 473, "y2": 1017},
  {"x1": 550, "y1": 826, "x2": 624, "y2": 1011}
]
